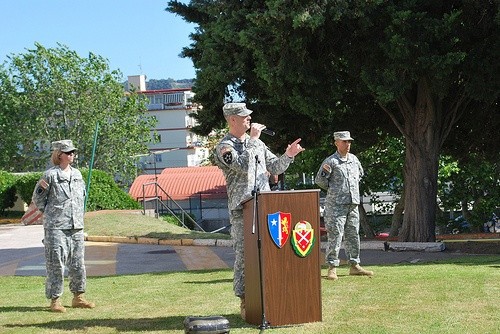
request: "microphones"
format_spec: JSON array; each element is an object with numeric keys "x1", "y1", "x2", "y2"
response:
[{"x1": 261, "y1": 129, "x2": 275, "y2": 136}]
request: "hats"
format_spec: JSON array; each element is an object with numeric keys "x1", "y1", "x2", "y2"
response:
[
  {"x1": 222, "y1": 103, "x2": 253, "y2": 117},
  {"x1": 333, "y1": 131, "x2": 354, "y2": 141},
  {"x1": 52, "y1": 139, "x2": 78, "y2": 153}
]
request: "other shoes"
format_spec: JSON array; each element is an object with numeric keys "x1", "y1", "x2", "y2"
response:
[
  {"x1": 349, "y1": 263, "x2": 373, "y2": 275},
  {"x1": 71, "y1": 293, "x2": 95, "y2": 308},
  {"x1": 327, "y1": 265, "x2": 337, "y2": 280},
  {"x1": 239, "y1": 297, "x2": 246, "y2": 321},
  {"x1": 50, "y1": 297, "x2": 66, "y2": 313}
]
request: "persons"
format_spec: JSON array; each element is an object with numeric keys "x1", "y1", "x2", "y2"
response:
[
  {"x1": 215, "y1": 103, "x2": 305, "y2": 320},
  {"x1": 315, "y1": 131, "x2": 374, "y2": 279},
  {"x1": 33, "y1": 140, "x2": 95, "y2": 313}
]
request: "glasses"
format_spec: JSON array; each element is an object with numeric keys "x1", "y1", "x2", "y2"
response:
[{"x1": 63, "y1": 150, "x2": 75, "y2": 155}]
